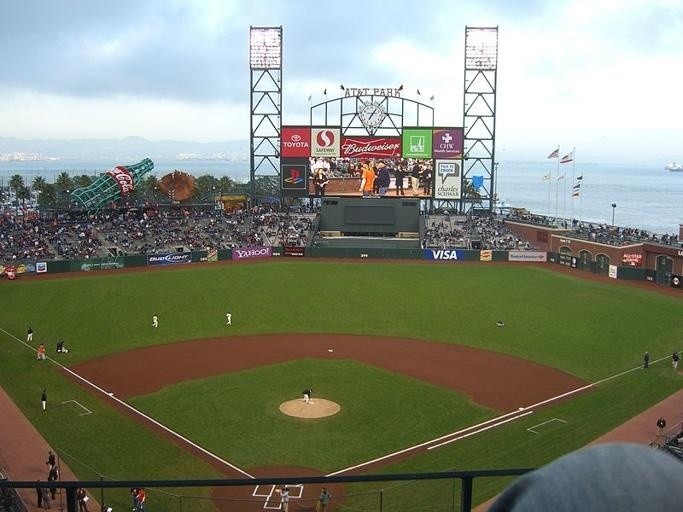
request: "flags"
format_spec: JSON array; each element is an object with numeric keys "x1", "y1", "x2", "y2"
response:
[
  {"x1": 559, "y1": 152, "x2": 573, "y2": 163},
  {"x1": 570, "y1": 177, "x2": 582, "y2": 196},
  {"x1": 547, "y1": 149, "x2": 559, "y2": 158}
]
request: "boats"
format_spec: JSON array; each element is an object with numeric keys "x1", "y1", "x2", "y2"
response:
[{"x1": 664, "y1": 160, "x2": 683, "y2": 172}]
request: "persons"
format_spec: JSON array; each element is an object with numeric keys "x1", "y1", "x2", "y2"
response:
[
  {"x1": 36, "y1": 451, "x2": 59, "y2": 510},
  {"x1": 319, "y1": 487, "x2": 330, "y2": 512},
  {"x1": 225, "y1": 312, "x2": 232, "y2": 328},
  {"x1": 56, "y1": 340, "x2": 64, "y2": 352},
  {"x1": 643, "y1": 351, "x2": 650, "y2": 367},
  {"x1": 152, "y1": 314, "x2": 158, "y2": 328},
  {"x1": 422, "y1": 207, "x2": 679, "y2": 252},
  {"x1": 308, "y1": 156, "x2": 433, "y2": 196},
  {"x1": 672, "y1": 351, "x2": 679, "y2": 369},
  {"x1": 27, "y1": 326, "x2": 34, "y2": 342},
  {"x1": 657, "y1": 415, "x2": 666, "y2": 435},
  {"x1": 77, "y1": 488, "x2": 89, "y2": 512},
  {"x1": 486, "y1": 441, "x2": 683, "y2": 512},
  {"x1": 303, "y1": 389, "x2": 312, "y2": 404},
  {"x1": 37, "y1": 343, "x2": 47, "y2": 360},
  {"x1": 131, "y1": 487, "x2": 145, "y2": 512},
  {"x1": 1, "y1": 204, "x2": 320, "y2": 263},
  {"x1": 280, "y1": 487, "x2": 289, "y2": 512},
  {"x1": 41, "y1": 389, "x2": 47, "y2": 411}
]
